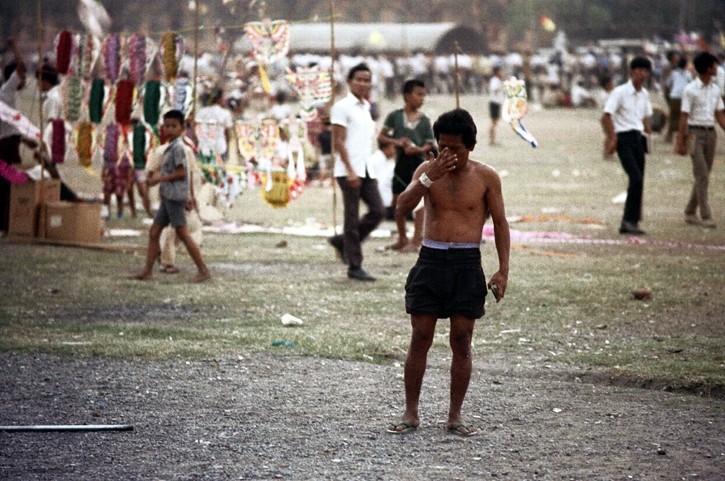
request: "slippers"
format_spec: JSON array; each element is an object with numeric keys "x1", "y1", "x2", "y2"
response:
[
  {"x1": 446, "y1": 419, "x2": 478, "y2": 436},
  {"x1": 386, "y1": 417, "x2": 418, "y2": 434}
]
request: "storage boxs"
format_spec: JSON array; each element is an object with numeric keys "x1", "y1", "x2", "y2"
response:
[
  {"x1": 39, "y1": 200, "x2": 101, "y2": 245},
  {"x1": 9, "y1": 179, "x2": 61, "y2": 238}
]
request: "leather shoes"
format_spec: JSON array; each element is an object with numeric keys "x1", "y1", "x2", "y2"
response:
[
  {"x1": 620, "y1": 225, "x2": 628, "y2": 234},
  {"x1": 328, "y1": 233, "x2": 351, "y2": 266},
  {"x1": 623, "y1": 221, "x2": 645, "y2": 235},
  {"x1": 346, "y1": 264, "x2": 377, "y2": 282}
]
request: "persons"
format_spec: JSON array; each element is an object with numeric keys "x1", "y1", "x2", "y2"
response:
[
  {"x1": 33, "y1": 61, "x2": 68, "y2": 176},
  {"x1": 128, "y1": 109, "x2": 214, "y2": 283},
  {"x1": 598, "y1": 55, "x2": 654, "y2": 236},
  {"x1": 378, "y1": 79, "x2": 436, "y2": 257},
  {"x1": 0, "y1": 36, "x2": 29, "y2": 239},
  {"x1": 143, "y1": 131, "x2": 201, "y2": 271},
  {"x1": 178, "y1": 41, "x2": 725, "y2": 147},
  {"x1": 385, "y1": 108, "x2": 512, "y2": 437},
  {"x1": 328, "y1": 63, "x2": 389, "y2": 284},
  {"x1": 675, "y1": 51, "x2": 725, "y2": 227}
]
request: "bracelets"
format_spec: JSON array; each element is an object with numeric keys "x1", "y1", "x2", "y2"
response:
[{"x1": 418, "y1": 173, "x2": 434, "y2": 189}]
